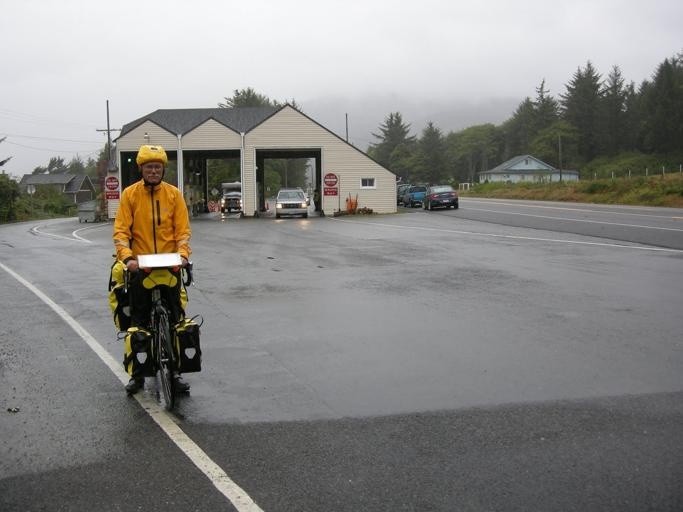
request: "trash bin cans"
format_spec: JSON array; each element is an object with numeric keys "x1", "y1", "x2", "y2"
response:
[{"x1": 78, "y1": 200, "x2": 100, "y2": 223}]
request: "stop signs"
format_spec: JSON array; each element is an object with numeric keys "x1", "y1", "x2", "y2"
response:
[
  {"x1": 105, "y1": 176, "x2": 119, "y2": 190},
  {"x1": 324, "y1": 172, "x2": 338, "y2": 186}
]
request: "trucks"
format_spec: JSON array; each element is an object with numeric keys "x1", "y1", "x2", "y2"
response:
[{"x1": 218, "y1": 181, "x2": 242, "y2": 213}]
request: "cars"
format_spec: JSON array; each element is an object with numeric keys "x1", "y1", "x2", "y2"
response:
[
  {"x1": 275, "y1": 188, "x2": 310, "y2": 219},
  {"x1": 397, "y1": 184, "x2": 459, "y2": 211}
]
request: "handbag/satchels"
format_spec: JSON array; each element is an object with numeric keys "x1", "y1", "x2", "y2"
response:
[
  {"x1": 106, "y1": 255, "x2": 189, "y2": 331},
  {"x1": 120, "y1": 316, "x2": 203, "y2": 377}
]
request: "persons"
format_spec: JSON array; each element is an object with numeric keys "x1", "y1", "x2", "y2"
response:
[{"x1": 110, "y1": 143, "x2": 193, "y2": 394}]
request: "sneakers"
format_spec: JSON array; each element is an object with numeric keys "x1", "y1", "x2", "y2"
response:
[
  {"x1": 175, "y1": 372, "x2": 191, "y2": 391},
  {"x1": 124, "y1": 374, "x2": 146, "y2": 394}
]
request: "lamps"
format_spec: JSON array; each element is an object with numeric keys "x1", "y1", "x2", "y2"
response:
[{"x1": 144, "y1": 133, "x2": 148, "y2": 139}]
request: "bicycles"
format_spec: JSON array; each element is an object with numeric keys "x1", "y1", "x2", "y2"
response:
[{"x1": 112, "y1": 253, "x2": 194, "y2": 412}]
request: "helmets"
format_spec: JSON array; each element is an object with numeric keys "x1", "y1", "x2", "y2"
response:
[{"x1": 133, "y1": 144, "x2": 169, "y2": 167}]
